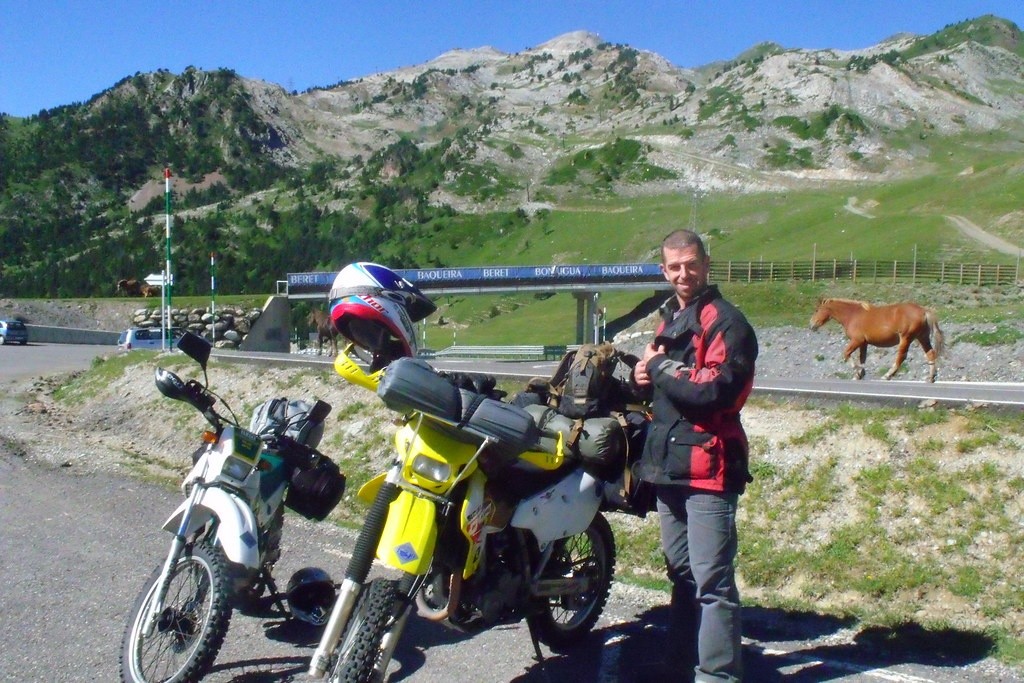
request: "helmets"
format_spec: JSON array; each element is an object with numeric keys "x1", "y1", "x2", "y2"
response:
[
  {"x1": 287, "y1": 566, "x2": 336, "y2": 626},
  {"x1": 329, "y1": 262, "x2": 437, "y2": 360}
]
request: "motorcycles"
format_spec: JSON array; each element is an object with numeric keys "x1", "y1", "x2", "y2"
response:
[
  {"x1": 117, "y1": 332, "x2": 347, "y2": 683},
  {"x1": 308, "y1": 330, "x2": 617, "y2": 683}
]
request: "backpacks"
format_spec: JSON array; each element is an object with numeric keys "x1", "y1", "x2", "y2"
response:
[{"x1": 529, "y1": 341, "x2": 640, "y2": 419}]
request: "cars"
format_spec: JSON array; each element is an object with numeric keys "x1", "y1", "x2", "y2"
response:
[
  {"x1": 0, "y1": 320, "x2": 28, "y2": 345},
  {"x1": 115, "y1": 329, "x2": 213, "y2": 355}
]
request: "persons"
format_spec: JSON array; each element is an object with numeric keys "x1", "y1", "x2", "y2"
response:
[{"x1": 629, "y1": 229, "x2": 758, "y2": 683}]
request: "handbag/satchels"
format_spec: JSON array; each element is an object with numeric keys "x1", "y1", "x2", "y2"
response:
[{"x1": 283, "y1": 453, "x2": 347, "y2": 521}]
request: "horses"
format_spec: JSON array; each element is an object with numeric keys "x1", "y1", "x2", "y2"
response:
[
  {"x1": 309, "y1": 308, "x2": 339, "y2": 357},
  {"x1": 809, "y1": 296, "x2": 943, "y2": 383}
]
